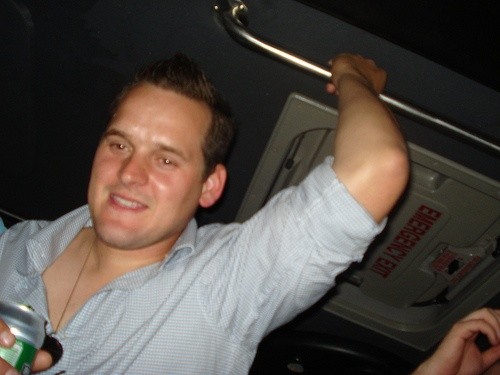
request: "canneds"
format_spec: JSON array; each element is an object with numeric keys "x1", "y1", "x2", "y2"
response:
[{"x1": 0, "y1": 300, "x2": 49, "y2": 375}]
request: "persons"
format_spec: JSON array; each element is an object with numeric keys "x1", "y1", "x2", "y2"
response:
[
  {"x1": 0, "y1": 46, "x2": 412, "y2": 375},
  {"x1": 412, "y1": 305, "x2": 499, "y2": 374}
]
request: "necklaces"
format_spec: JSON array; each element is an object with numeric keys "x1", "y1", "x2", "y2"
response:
[{"x1": 53, "y1": 239, "x2": 96, "y2": 334}]
